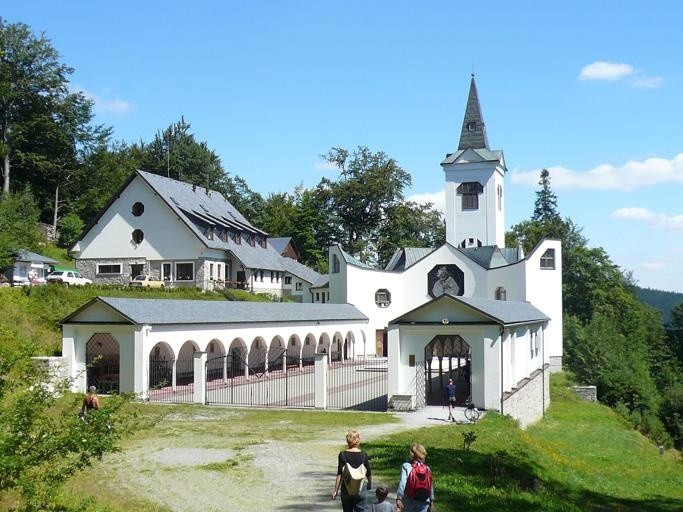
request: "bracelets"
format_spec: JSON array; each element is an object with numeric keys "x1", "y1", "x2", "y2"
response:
[{"x1": 396, "y1": 499, "x2": 401, "y2": 500}]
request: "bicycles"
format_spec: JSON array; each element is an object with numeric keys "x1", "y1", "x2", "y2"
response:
[{"x1": 464, "y1": 397, "x2": 480, "y2": 421}]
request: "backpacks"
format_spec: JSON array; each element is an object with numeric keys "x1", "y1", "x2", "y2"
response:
[
  {"x1": 405, "y1": 461, "x2": 432, "y2": 501},
  {"x1": 341, "y1": 451, "x2": 368, "y2": 497}
]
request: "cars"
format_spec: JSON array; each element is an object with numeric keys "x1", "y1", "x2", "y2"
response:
[
  {"x1": 129, "y1": 275, "x2": 166, "y2": 288},
  {"x1": 45, "y1": 271, "x2": 92, "y2": 288}
]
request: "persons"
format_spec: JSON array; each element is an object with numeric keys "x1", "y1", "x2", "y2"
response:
[
  {"x1": 82, "y1": 386, "x2": 99, "y2": 412},
  {"x1": 372, "y1": 486, "x2": 400, "y2": 512},
  {"x1": 445, "y1": 379, "x2": 456, "y2": 422},
  {"x1": 396, "y1": 443, "x2": 434, "y2": 512},
  {"x1": 332, "y1": 431, "x2": 372, "y2": 512}
]
